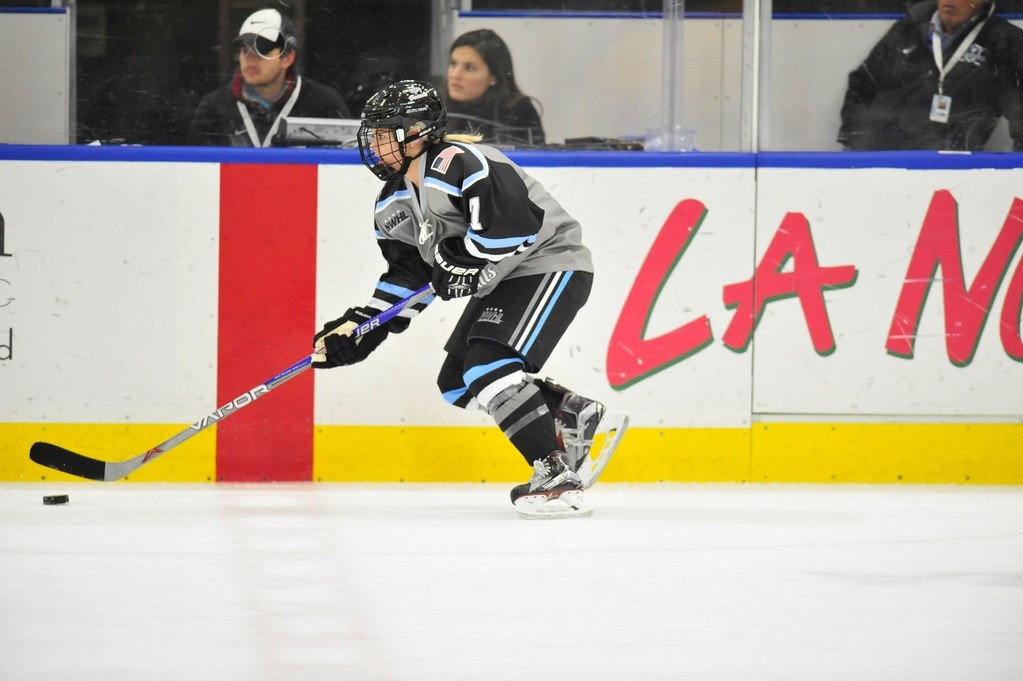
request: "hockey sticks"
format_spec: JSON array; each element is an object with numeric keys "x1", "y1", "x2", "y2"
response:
[{"x1": 28, "y1": 280, "x2": 437, "y2": 482}]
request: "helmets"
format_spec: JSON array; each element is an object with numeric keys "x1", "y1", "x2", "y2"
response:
[{"x1": 357, "y1": 79, "x2": 448, "y2": 181}]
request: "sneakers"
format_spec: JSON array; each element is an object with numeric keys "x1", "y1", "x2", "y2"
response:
[
  {"x1": 555, "y1": 392, "x2": 629, "y2": 490},
  {"x1": 510, "y1": 450, "x2": 594, "y2": 520}
]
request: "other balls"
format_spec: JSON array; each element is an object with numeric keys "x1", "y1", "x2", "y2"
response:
[{"x1": 42, "y1": 494, "x2": 70, "y2": 506}]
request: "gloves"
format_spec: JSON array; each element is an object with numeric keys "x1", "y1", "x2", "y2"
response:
[
  {"x1": 311, "y1": 307, "x2": 389, "y2": 369},
  {"x1": 431, "y1": 236, "x2": 489, "y2": 301}
]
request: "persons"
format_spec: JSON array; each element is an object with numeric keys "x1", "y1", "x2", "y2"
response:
[
  {"x1": 310, "y1": 80, "x2": 627, "y2": 519},
  {"x1": 836, "y1": 1, "x2": 1023, "y2": 155},
  {"x1": 182, "y1": 8, "x2": 350, "y2": 148},
  {"x1": 436, "y1": 29, "x2": 546, "y2": 144}
]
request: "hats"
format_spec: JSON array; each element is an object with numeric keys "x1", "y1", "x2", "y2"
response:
[{"x1": 231, "y1": 7, "x2": 298, "y2": 49}]
